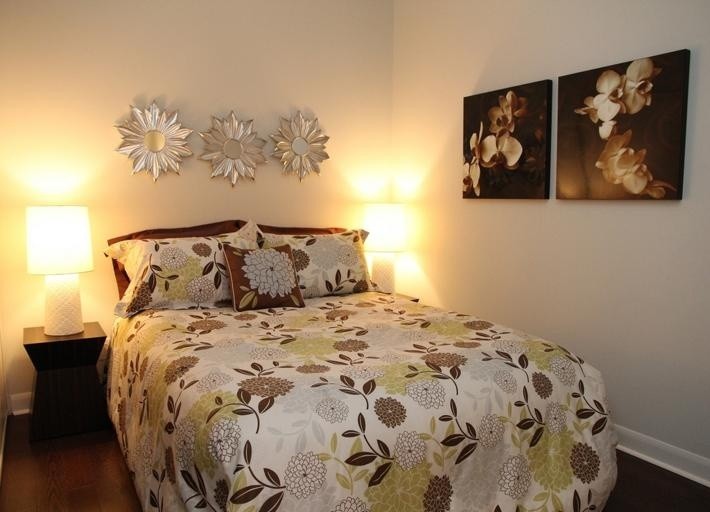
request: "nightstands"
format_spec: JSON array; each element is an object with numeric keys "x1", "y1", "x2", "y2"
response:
[
  {"x1": 375, "y1": 286, "x2": 417, "y2": 304},
  {"x1": 21, "y1": 322, "x2": 109, "y2": 441}
]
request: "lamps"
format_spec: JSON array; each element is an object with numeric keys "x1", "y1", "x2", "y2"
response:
[
  {"x1": 24, "y1": 203, "x2": 95, "y2": 336},
  {"x1": 356, "y1": 209, "x2": 410, "y2": 296}
]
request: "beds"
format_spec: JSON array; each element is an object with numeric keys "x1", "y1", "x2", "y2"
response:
[{"x1": 103, "y1": 218, "x2": 619, "y2": 512}]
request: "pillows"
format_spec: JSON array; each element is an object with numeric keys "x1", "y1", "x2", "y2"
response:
[{"x1": 107, "y1": 218, "x2": 379, "y2": 318}]
